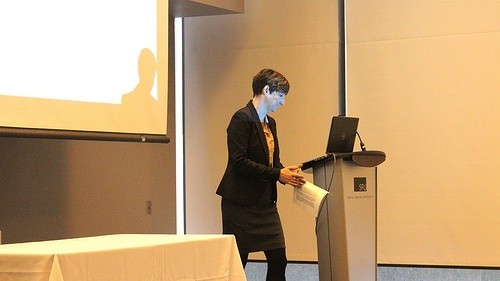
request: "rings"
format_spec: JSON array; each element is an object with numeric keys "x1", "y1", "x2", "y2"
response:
[{"x1": 293, "y1": 176, "x2": 295, "y2": 180}]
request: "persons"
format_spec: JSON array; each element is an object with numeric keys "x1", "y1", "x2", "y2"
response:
[{"x1": 216, "y1": 68, "x2": 306, "y2": 281}]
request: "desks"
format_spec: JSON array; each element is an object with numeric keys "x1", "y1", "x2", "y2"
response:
[{"x1": 1, "y1": 234, "x2": 247, "y2": 281}]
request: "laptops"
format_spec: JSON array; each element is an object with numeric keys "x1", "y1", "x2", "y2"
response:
[{"x1": 303, "y1": 116, "x2": 359, "y2": 166}]
